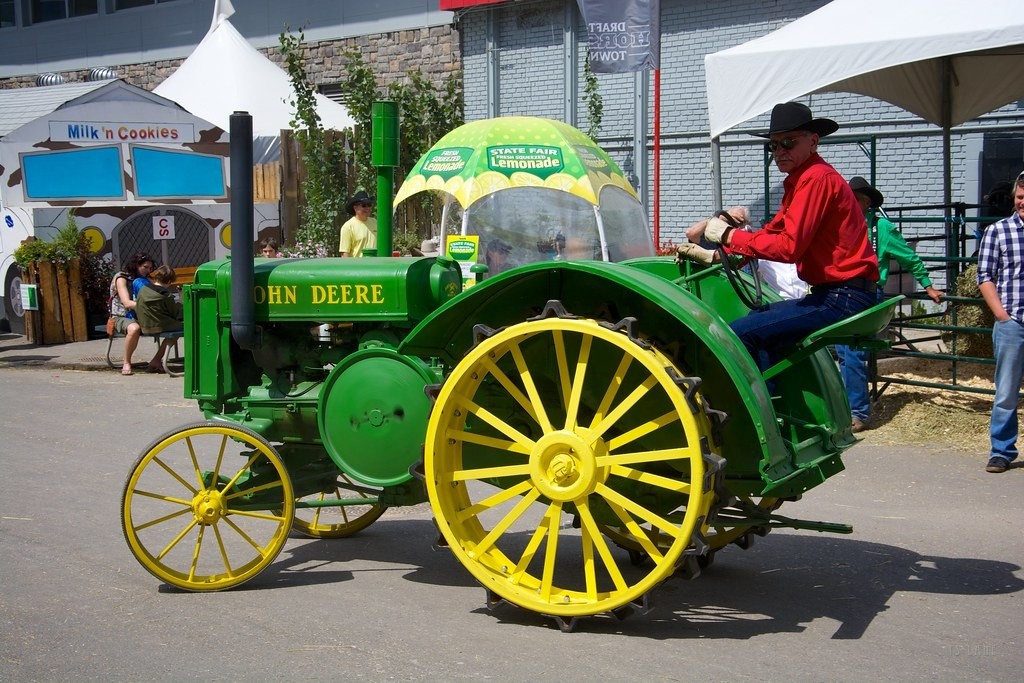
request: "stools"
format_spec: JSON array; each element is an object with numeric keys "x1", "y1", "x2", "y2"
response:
[{"x1": 775, "y1": 294, "x2": 907, "y2": 450}]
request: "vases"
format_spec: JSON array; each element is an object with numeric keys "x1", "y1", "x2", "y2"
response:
[{"x1": 21, "y1": 256, "x2": 89, "y2": 346}]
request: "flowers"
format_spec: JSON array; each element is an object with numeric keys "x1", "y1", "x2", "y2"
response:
[{"x1": 13, "y1": 207, "x2": 84, "y2": 274}]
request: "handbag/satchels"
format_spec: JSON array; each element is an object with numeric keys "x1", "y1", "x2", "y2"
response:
[{"x1": 107, "y1": 317, "x2": 116, "y2": 336}]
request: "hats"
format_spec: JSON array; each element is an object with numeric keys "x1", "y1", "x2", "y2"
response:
[
  {"x1": 346, "y1": 191, "x2": 375, "y2": 213},
  {"x1": 849, "y1": 176, "x2": 884, "y2": 209},
  {"x1": 748, "y1": 102, "x2": 839, "y2": 138},
  {"x1": 487, "y1": 238, "x2": 513, "y2": 251}
]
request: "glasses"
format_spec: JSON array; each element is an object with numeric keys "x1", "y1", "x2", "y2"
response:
[
  {"x1": 499, "y1": 249, "x2": 510, "y2": 256},
  {"x1": 765, "y1": 133, "x2": 810, "y2": 152},
  {"x1": 358, "y1": 202, "x2": 372, "y2": 208}
]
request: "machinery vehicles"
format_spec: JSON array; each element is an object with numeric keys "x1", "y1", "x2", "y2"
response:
[{"x1": 121, "y1": 101, "x2": 906, "y2": 635}]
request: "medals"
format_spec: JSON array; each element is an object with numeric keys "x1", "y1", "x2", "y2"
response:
[{"x1": 704, "y1": 0, "x2": 1024, "y2": 307}]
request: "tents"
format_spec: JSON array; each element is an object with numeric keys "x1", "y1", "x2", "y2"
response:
[
  {"x1": 151, "y1": 0, "x2": 366, "y2": 244},
  {"x1": 392, "y1": 115, "x2": 656, "y2": 294}
]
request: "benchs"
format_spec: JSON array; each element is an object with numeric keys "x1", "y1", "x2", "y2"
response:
[{"x1": 106, "y1": 330, "x2": 184, "y2": 377}]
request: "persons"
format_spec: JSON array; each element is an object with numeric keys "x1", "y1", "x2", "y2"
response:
[
  {"x1": 338, "y1": 191, "x2": 378, "y2": 258},
  {"x1": 685, "y1": 206, "x2": 758, "y2": 278},
  {"x1": 974, "y1": 174, "x2": 1024, "y2": 472},
  {"x1": 135, "y1": 265, "x2": 184, "y2": 335},
  {"x1": 758, "y1": 259, "x2": 809, "y2": 302},
  {"x1": 109, "y1": 252, "x2": 179, "y2": 375},
  {"x1": 479, "y1": 234, "x2": 518, "y2": 281},
  {"x1": 675, "y1": 102, "x2": 879, "y2": 413},
  {"x1": 834, "y1": 176, "x2": 946, "y2": 433},
  {"x1": 259, "y1": 240, "x2": 279, "y2": 259}
]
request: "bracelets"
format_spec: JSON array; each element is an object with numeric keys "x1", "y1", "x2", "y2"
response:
[{"x1": 721, "y1": 225, "x2": 734, "y2": 246}]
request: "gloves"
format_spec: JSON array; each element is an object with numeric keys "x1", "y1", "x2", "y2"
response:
[
  {"x1": 674, "y1": 243, "x2": 715, "y2": 268},
  {"x1": 705, "y1": 216, "x2": 733, "y2": 244}
]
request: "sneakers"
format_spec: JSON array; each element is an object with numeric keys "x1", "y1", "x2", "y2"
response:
[
  {"x1": 985, "y1": 457, "x2": 1010, "y2": 472},
  {"x1": 852, "y1": 418, "x2": 865, "y2": 432}
]
